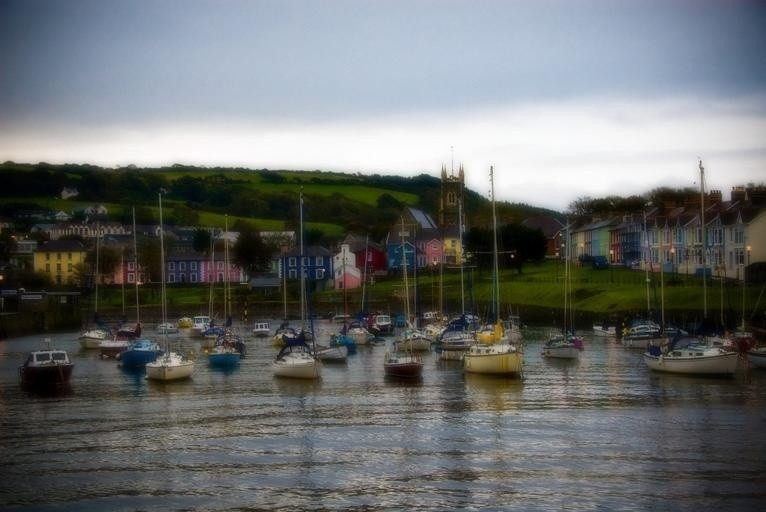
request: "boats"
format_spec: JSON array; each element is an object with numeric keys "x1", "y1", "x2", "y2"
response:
[{"x1": 19, "y1": 338, "x2": 75, "y2": 392}]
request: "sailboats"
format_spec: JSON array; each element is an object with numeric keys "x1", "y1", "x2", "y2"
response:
[
  {"x1": 78, "y1": 159, "x2": 766, "y2": 375},
  {"x1": 382, "y1": 215, "x2": 425, "y2": 379},
  {"x1": 642, "y1": 230, "x2": 739, "y2": 374},
  {"x1": 463, "y1": 165, "x2": 524, "y2": 375},
  {"x1": 144, "y1": 193, "x2": 196, "y2": 381},
  {"x1": 271, "y1": 183, "x2": 326, "y2": 380}
]
